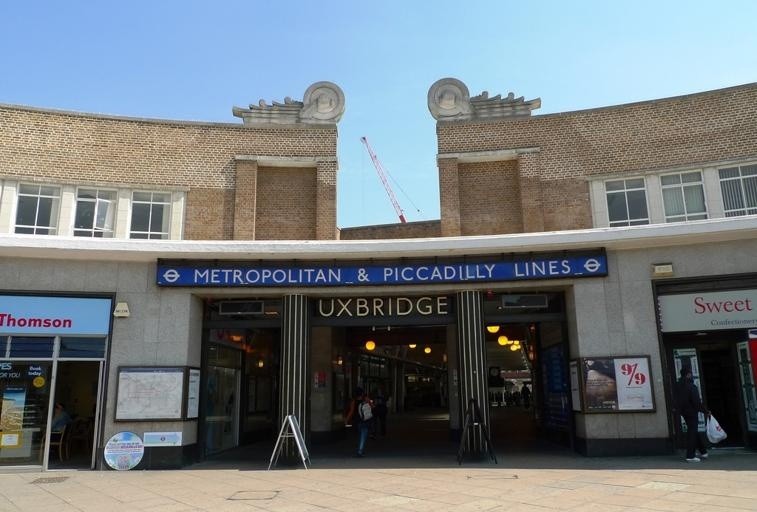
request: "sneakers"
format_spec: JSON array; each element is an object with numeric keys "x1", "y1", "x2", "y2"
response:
[
  {"x1": 686, "y1": 457, "x2": 699, "y2": 462},
  {"x1": 701, "y1": 453, "x2": 708, "y2": 458}
]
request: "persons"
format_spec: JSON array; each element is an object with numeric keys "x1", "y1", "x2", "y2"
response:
[
  {"x1": 372, "y1": 388, "x2": 388, "y2": 440},
  {"x1": 49, "y1": 402, "x2": 74, "y2": 454},
  {"x1": 343, "y1": 385, "x2": 375, "y2": 458},
  {"x1": 670, "y1": 367, "x2": 712, "y2": 464},
  {"x1": 520, "y1": 383, "x2": 529, "y2": 410}
]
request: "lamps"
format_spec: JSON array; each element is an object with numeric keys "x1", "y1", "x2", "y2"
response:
[
  {"x1": 486, "y1": 325, "x2": 500, "y2": 333},
  {"x1": 422, "y1": 347, "x2": 432, "y2": 354},
  {"x1": 497, "y1": 336, "x2": 508, "y2": 346},
  {"x1": 409, "y1": 344, "x2": 417, "y2": 348},
  {"x1": 365, "y1": 340, "x2": 377, "y2": 351}
]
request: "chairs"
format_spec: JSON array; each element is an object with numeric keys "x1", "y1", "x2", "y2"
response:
[{"x1": 35, "y1": 419, "x2": 72, "y2": 462}]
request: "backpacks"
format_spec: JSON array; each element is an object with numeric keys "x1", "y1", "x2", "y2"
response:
[{"x1": 358, "y1": 401, "x2": 375, "y2": 422}]
